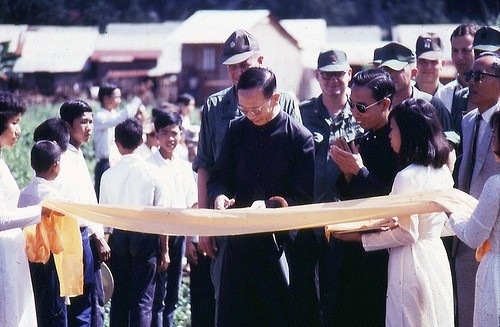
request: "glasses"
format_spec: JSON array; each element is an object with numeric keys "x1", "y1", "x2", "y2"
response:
[
  {"x1": 347, "y1": 93, "x2": 391, "y2": 113},
  {"x1": 319, "y1": 72, "x2": 346, "y2": 80},
  {"x1": 464, "y1": 70, "x2": 500, "y2": 82},
  {"x1": 237, "y1": 93, "x2": 276, "y2": 116}
]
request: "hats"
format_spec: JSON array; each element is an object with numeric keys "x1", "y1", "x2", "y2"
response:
[
  {"x1": 317, "y1": 50, "x2": 349, "y2": 72},
  {"x1": 471, "y1": 25, "x2": 500, "y2": 53},
  {"x1": 220, "y1": 29, "x2": 260, "y2": 65},
  {"x1": 369, "y1": 48, "x2": 382, "y2": 64},
  {"x1": 415, "y1": 32, "x2": 444, "y2": 60},
  {"x1": 377, "y1": 43, "x2": 417, "y2": 71}
]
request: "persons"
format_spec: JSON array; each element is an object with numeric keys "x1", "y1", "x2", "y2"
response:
[
  {"x1": 0, "y1": 90, "x2": 67, "y2": 327},
  {"x1": 15, "y1": 22, "x2": 500, "y2": 327}
]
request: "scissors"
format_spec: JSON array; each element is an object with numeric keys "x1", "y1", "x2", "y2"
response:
[{"x1": 216, "y1": 200, "x2": 231, "y2": 211}]
